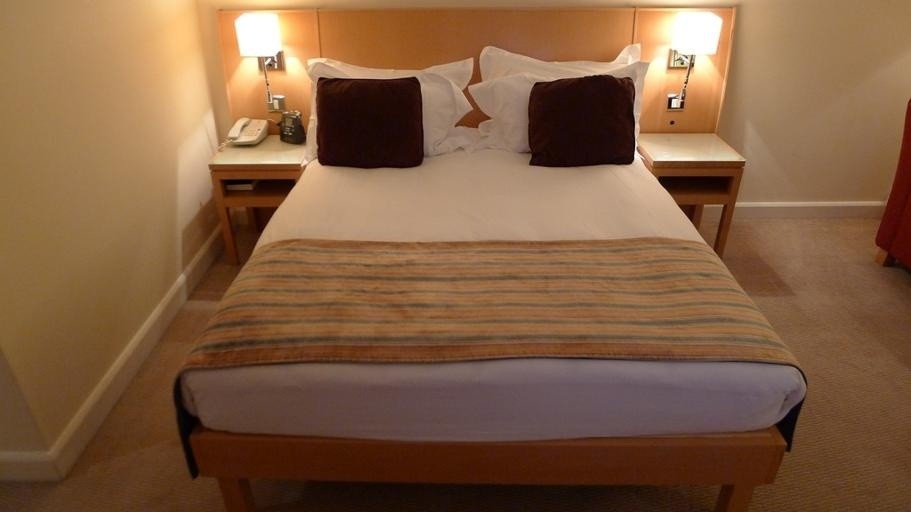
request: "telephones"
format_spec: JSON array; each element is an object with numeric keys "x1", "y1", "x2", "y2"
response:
[{"x1": 227, "y1": 117, "x2": 269, "y2": 146}]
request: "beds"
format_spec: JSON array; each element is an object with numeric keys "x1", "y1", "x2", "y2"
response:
[{"x1": 174, "y1": 8, "x2": 807, "y2": 512}]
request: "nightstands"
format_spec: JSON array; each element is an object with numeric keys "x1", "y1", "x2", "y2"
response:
[
  {"x1": 209, "y1": 133, "x2": 309, "y2": 266},
  {"x1": 636, "y1": 133, "x2": 745, "y2": 257}
]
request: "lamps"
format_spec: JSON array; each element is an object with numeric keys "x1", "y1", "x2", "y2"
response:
[
  {"x1": 667, "y1": 12, "x2": 722, "y2": 111},
  {"x1": 234, "y1": 11, "x2": 284, "y2": 111}
]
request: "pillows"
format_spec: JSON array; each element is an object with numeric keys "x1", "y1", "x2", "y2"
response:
[
  {"x1": 301, "y1": 60, "x2": 475, "y2": 166},
  {"x1": 314, "y1": 74, "x2": 426, "y2": 168},
  {"x1": 477, "y1": 46, "x2": 649, "y2": 136},
  {"x1": 304, "y1": 55, "x2": 475, "y2": 90},
  {"x1": 526, "y1": 72, "x2": 639, "y2": 166},
  {"x1": 465, "y1": 60, "x2": 651, "y2": 154}
]
